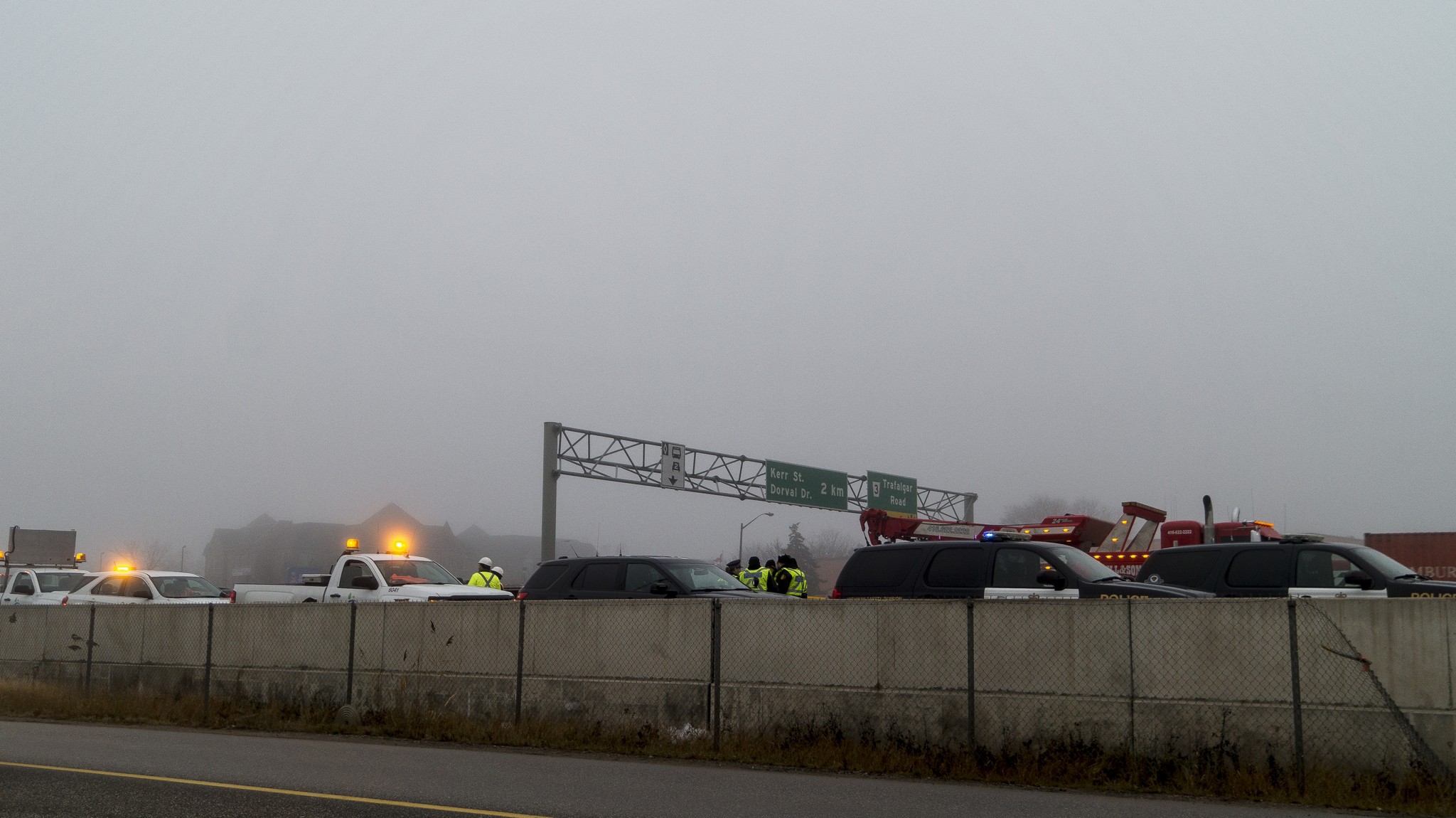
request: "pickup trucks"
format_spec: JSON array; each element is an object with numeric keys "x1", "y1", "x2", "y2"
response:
[
  {"x1": 1, "y1": 568, "x2": 92, "y2": 606},
  {"x1": 233, "y1": 552, "x2": 514, "y2": 601}
]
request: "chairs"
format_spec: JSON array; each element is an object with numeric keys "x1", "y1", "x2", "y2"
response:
[
  {"x1": 1008, "y1": 563, "x2": 1028, "y2": 588},
  {"x1": 59, "y1": 576, "x2": 78, "y2": 593},
  {"x1": 994, "y1": 567, "x2": 1013, "y2": 588},
  {"x1": 102, "y1": 583, "x2": 114, "y2": 596},
  {"x1": 134, "y1": 590, "x2": 150, "y2": 600},
  {"x1": 341, "y1": 566, "x2": 362, "y2": 589},
  {"x1": 165, "y1": 583, "x2": 175, "y2": 592},
  {"x1": 385, "y1": 567, "x2": 404, "y2": 585},
  {"x1": 21, "y1": 578, "x2": 41, "y2": 591}
]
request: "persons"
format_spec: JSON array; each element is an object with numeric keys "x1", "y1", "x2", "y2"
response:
[
  {"x1": 466, "y1": 557, "x2": 503, "y2": 591},
  {"x1": 791, "y1": 558, "x2": 807, "y2": 598},
  {"x1": 739, "y1": 556, "x2": 779, "y2": 593},
  {"x1": 490, "y1": 566, "x2": 503, "y2": 591},
  {"x1": 774, "y1": 554, "x2": 802, "y2": 598},
  {"x1": 717, "y1": 559, "x2": 743, "y2": 589},
  {"x1": 764, "y1": 559, "x2": 778, "y2": 578}
]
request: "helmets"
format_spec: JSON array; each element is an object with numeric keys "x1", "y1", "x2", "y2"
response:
[
  {"x1": 477, "y1": 557, "x2": 492, "y2": 567},
  {"x1": 490, "y1": 566, "x2": 504, "y2": 578}
]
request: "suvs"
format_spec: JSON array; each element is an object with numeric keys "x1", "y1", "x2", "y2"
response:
[
  {"x1": 1135, "y1": 541, "x2": 1456, "y2": 598},
  {"x1": 61, "y1": 570, "x2": 235, "y2": 605},
  {"x1": 829, "y1": 537, "x2": 1216, "y2": 597},
  {"x1": 518, "y1": 555, "x2": 806, "y2": 600}
]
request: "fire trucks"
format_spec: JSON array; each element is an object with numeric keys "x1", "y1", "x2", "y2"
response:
[{"x1": 858, "y1": 507, "x2": 1282, "y2": 579}]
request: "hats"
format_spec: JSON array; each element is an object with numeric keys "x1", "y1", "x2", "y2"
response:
[
  {"x1": 776, "y1": 554, "x2": 798, "y2": 566},
  {"x1": 747, "y1": 556, "x2": 760, "y2": 565},
  {"x1": 726, "y1": 559, "x2": 744, "y2": 568},
  {"x1": 765, "y1": 559, "x2": 776, "y2": 570}
]
